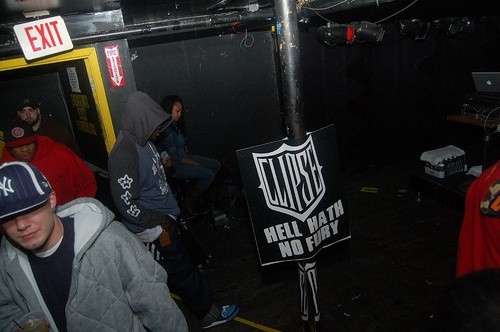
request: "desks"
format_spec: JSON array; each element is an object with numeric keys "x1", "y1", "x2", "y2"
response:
[{"x1": 446, "y1": 115, "x2": 500, "y2": 163}]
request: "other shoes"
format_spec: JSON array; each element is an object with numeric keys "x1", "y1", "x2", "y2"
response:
[{"x1": 202, "y1": 303, "x2": 238, "y2": 328}]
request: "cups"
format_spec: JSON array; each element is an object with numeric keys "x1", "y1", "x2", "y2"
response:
[{"x1": 16, "y1": 310, "x2": 50, "y2": 332}]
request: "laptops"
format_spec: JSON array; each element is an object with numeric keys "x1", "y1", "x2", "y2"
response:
[{"x1": 472, "y1": 72, "x2": 500, "y2": 96}]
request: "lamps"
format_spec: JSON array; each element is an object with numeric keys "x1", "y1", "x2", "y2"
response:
[
  {"x1": 351, "y1": 22, "x2": 384, "y2": 42},
  {"x1": 316, "y1": 21, "x2": 354, "y2": 50},
  {"x1": 396, "y1": 18, "x2": 430, "y2": 40},
  {"x1": 433, "y1": 17, "x2": 473, "y2": 33}
]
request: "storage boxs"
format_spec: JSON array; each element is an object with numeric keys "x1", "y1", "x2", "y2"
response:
[{"x1": 420, "y1": 145, "x2": 467, "y2": 179}]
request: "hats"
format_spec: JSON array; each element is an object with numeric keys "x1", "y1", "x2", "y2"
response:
[
  {"x1": 1, "y1": 160, "x2": 52, "y2": 220},
  {"x1": 4, "y1": 118, "x2": 37, "y2": 148},
  {"x1": 15, "y1": 94, "x2": 41, "y2": 111}
]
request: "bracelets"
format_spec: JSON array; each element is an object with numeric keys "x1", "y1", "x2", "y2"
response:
[{"x1": 165, "y1": 219, "x2": 175, "y2": 233}]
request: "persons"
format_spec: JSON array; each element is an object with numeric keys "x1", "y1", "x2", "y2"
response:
[
  {"x1": 0, "y1": 161, "x2": 189, "y2": 332},
  {"x1": 0, "y1": 90, "x2": 238, "y2": 329}
]
